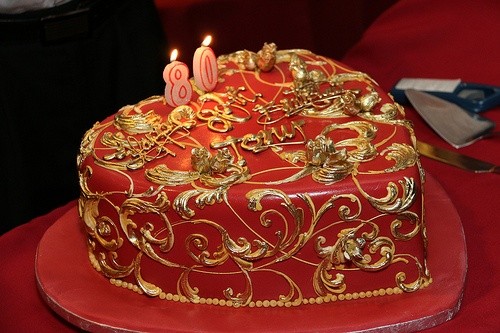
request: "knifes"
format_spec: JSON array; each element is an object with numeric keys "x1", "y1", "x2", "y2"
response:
[{"x1": 416, "y1": 139, "x2": 499, "y2": 176}]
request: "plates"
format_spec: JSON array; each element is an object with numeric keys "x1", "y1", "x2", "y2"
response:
[{"x1": 33, "y1": 170, "x2": 469, "y2": 332}]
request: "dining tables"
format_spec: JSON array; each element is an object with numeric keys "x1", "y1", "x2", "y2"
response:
[{"x1": 1, "y1": 0, "x2": 500, "y2": 333}]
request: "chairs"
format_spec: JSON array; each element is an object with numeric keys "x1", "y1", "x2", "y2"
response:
[{"x1": 1, "y1": 1, "x2": 168, "y2": 235}]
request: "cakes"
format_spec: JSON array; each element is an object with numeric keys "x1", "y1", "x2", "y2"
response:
[{"x1": 76, "y1": 40, "x2": 432, "y2": 307}]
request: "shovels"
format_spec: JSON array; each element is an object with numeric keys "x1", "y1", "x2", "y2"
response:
[{"x1": 403, "y1": 88, "x2": 500, "y2": 149}]
